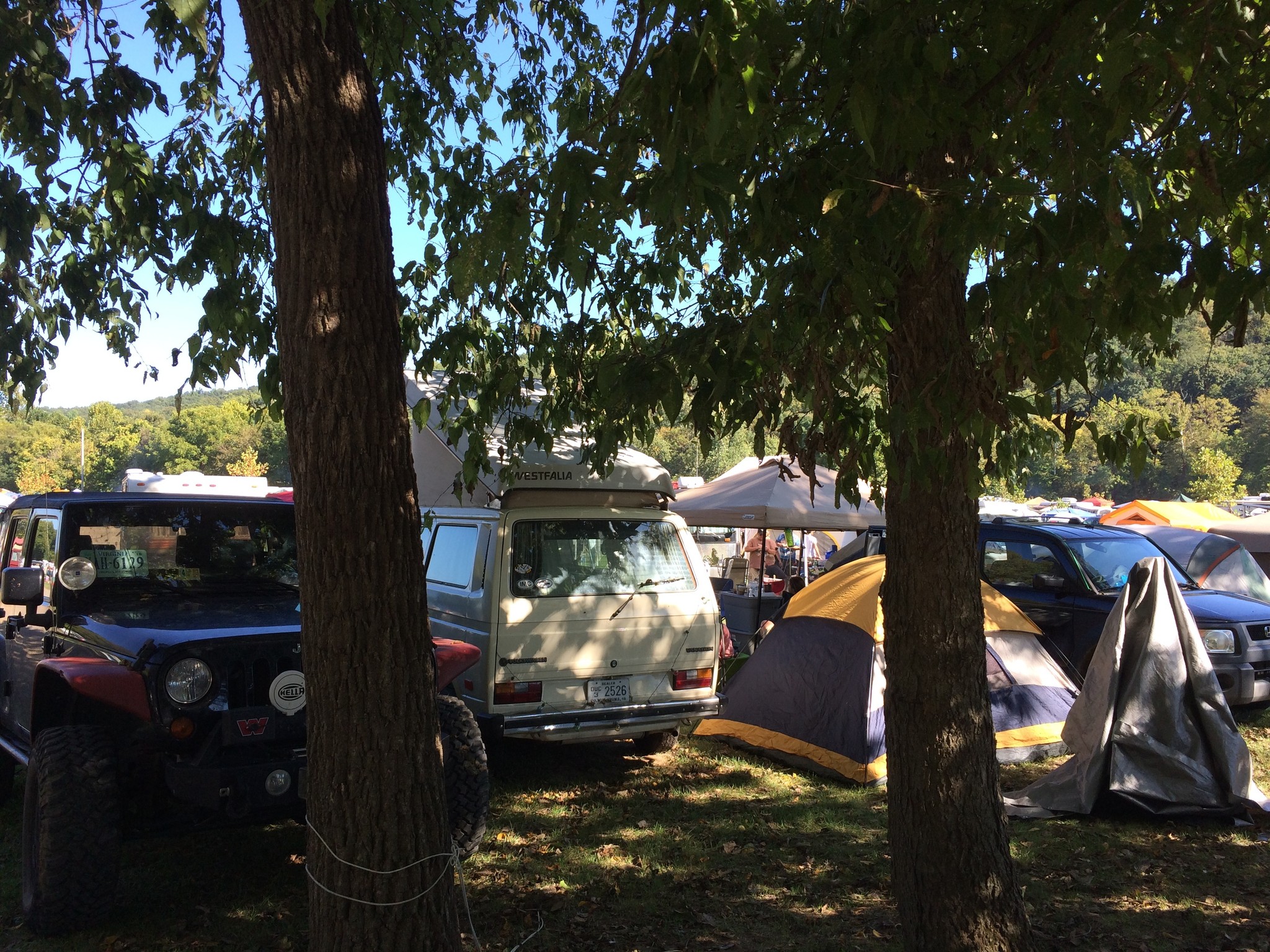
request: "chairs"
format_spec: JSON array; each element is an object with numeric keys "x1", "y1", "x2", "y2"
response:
[
  {"x1": 721, "y1": 557, "x2": 749, "y2": 592},
  {"x1": 987, "y1": 556, "x2": 1056, "y2": 585}
]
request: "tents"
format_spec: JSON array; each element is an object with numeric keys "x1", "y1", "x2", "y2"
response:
[{"x1": 669, "y1": 458, "x2": 1270, "y2": 831}]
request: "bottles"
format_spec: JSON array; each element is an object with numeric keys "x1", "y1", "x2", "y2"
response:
[
  {"x1": 814, "y1": 559, "x2": 818, "y2": 567},
  {"x1": 785, "y1": 527, "x2": 794, "y2": 547},
  {"x1": 747, "y1": 581, "x2": 758, "y2": 597}
]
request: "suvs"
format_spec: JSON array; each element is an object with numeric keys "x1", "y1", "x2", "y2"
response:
[
  {"x1": 974, "y1": 515, "x2": 1270, "y2": 719},
  {"x1": 0, "y1": 490, "x2": 488, "y2": 937}
]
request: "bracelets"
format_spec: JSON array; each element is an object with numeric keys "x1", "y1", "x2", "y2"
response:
[{"x1": 767, "y1": 548, "x2": 769, "y2": 551}]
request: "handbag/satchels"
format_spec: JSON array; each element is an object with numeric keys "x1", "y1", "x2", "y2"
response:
[{"x1": 730, "y1": 639, "x2": 740, "y2": 658}]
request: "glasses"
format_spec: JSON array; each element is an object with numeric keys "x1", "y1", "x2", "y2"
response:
[{"x1": 759, "y1": 533, "x2": 762, "y2": 536}]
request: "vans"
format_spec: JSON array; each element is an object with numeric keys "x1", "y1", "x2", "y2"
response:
[{"x1": 399, "y1": 366, "x2": 723, "y2": 772}]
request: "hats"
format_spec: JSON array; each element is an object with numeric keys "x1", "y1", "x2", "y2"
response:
[{"x1": 758, "y1": 529, "x2": 764, "y2": 533}]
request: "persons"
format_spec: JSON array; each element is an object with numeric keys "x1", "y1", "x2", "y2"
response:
[
  {"x1": 773, "y1": 541, "x2": 782, "y2": 566},
  {"x1": 745, "y1": 528, "x2": 776, "y2": 583},
  {"x1": 764, "y1": 555, "x2": 787, "y2": 579},
  {"x1": 796, "y1": 530, "x2": 821, "y2": 576},
  {"x1": 775, "y1": 530, "x2": 800, "y2": 576}
]
